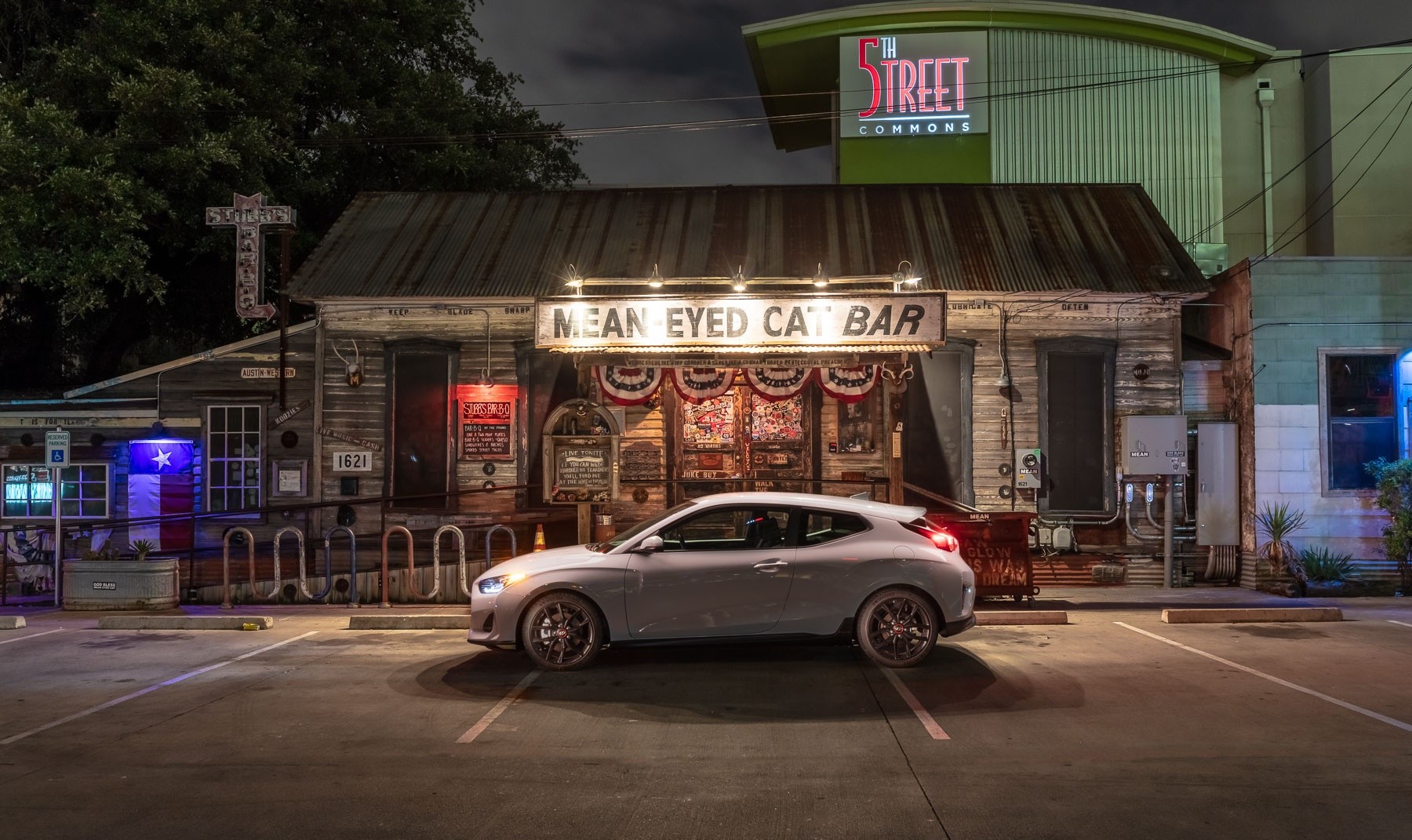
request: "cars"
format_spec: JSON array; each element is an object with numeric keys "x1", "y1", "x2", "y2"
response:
[{"x1": 465, "y1": 491, "x2": 977, "y2": 674}]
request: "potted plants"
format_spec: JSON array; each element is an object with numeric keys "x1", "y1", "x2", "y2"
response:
[{"x1": 56, "y1": 539, "x2": 181, "y2": 610}]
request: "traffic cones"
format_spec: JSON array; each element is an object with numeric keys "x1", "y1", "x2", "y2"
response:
[{"x1": 533, "y1": 524, "x2": 547, "y2": 553}]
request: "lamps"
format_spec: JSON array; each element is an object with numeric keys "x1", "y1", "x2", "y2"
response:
[
  {"x1": 812, "y1": 262, "x2": 830, "y2": 286},
  {"x1": 893, "y1": 260, "x2": 922, "y2": 286},
  {"x1": 993, "y1": 372, "x2": 1011, "y2": 390},
  {"x1": 564, "y1": 264, "x2": 584, "y2": 289},
  {"x1": 467, "y1": 367, "x2": 497, "y2": 399},
  {"x1": 648, "y1": 263, "x2": 665, "y2": 288},
  {"x1": 731, "y1": 263, "x2": 746, "y2": 291}
]
